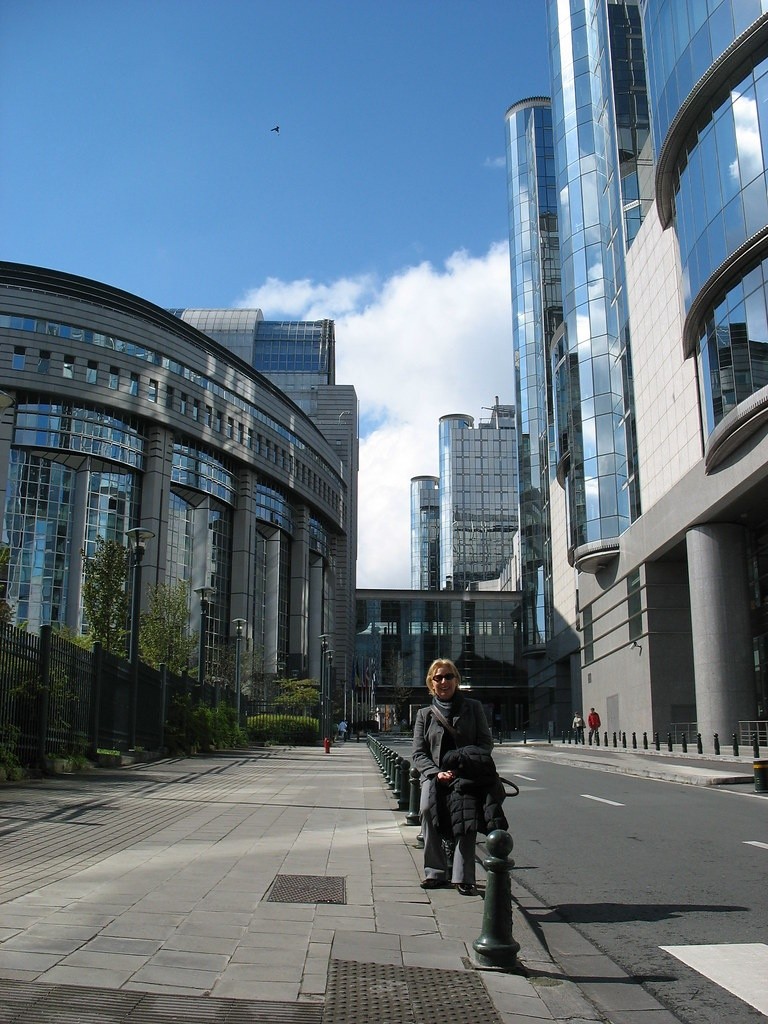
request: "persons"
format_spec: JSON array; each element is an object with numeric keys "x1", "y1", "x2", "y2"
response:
[
  {"x1": 338, "y1": 720, "x2": 351, "y2": 741},
  {"x1": 410, "y1": 658, "x2": 493, "y2": 896},
  {"x1": 588, "y1": 708, "x2": 601, "y2": 743},
  {"x1": 572, "y1": 712, "x2": 586, "y2": 743}
]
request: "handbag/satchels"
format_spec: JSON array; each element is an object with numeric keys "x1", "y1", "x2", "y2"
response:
[{"x1": 581, "y1": 717, "x2": 586, "y2": 728}]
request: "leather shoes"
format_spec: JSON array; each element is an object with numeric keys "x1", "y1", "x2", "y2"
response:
[
  {"x1": 420, "y1": 877, "x2": 446, "y2": 888},
  {"x1": 457, "y1": 882, "x2": 474, "y2": 896}
]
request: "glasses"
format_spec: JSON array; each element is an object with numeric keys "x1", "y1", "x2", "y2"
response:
[{"x1": 432, "y1": 673, "x2": 457, "y2": 682}]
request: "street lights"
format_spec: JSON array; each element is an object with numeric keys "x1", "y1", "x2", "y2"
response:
[
  {"x1": 231, "y1": 617, "x2": 249, "y2": 728},
  {"x1": 319, "y1": 633, "x2": 336, "y2": 742},
  {"x1": 123, "y1": 526, "x2": 157, "y2": 755}
]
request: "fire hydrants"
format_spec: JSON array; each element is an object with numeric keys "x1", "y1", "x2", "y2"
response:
[{"x1": 324, "y1": 738, "x2": 332, "y2": 753}]
